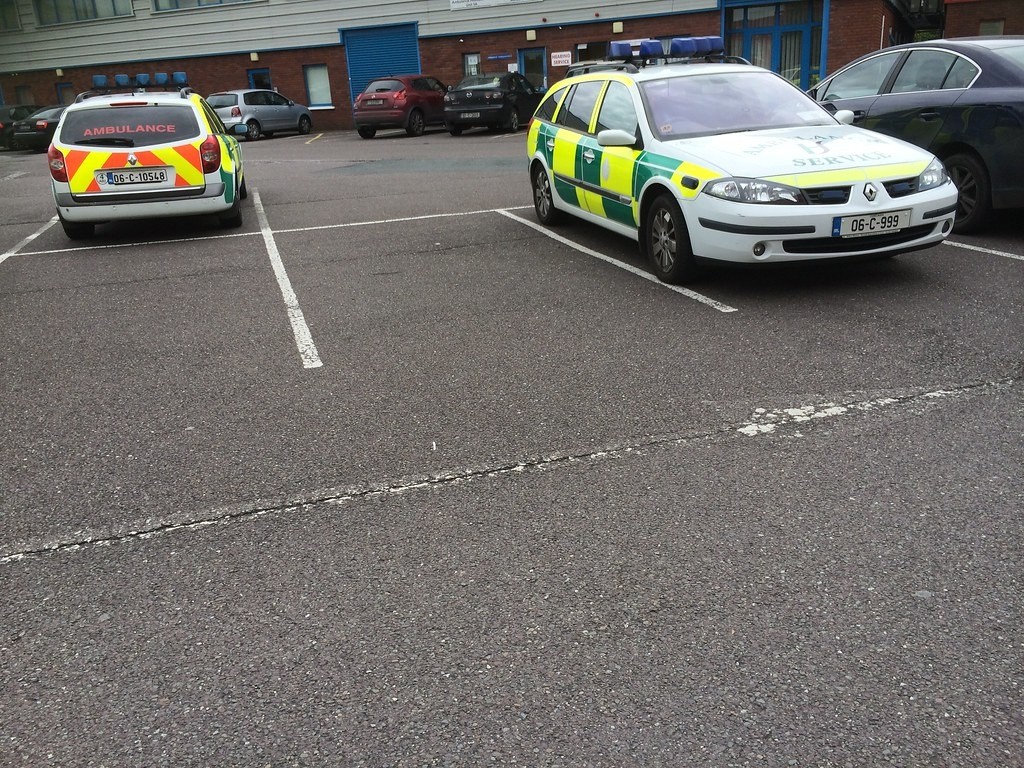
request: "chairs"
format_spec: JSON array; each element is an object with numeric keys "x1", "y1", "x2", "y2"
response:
[{"x1": 911, "y1": 59, "x2": 946, "y2": 90}]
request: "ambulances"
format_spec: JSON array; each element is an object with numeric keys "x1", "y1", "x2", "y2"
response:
[
  {"x1": 527, "y1": 35, "x2": 958, "y2": 285},
  {"x1": 48, "y1": 71, "x2": 247, "y2": 240}
]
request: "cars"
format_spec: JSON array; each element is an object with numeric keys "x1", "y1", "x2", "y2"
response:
[
  {"x1": 0, "y1": 105, "x2": 68, "y2": 152},
  {"x1": 352, "y1": 74, "x2": 452, "y2": 138},
  {"x1": 442, "y1": 72, "x2": 546, "y2": 136},
  {"x1": 206, "y1": 89, "x2": 313, "y2": 141},
  {"x1": 792, "y1": 34, "x2": 1024, "y2": 234}
]
